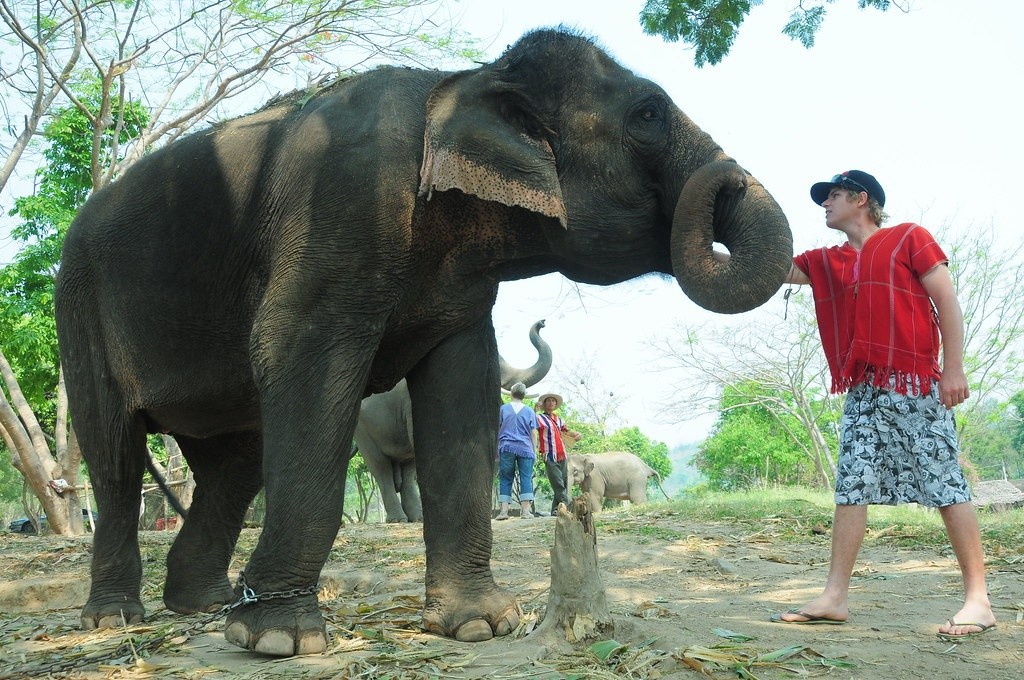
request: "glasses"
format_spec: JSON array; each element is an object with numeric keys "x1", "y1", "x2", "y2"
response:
[{"x1": 832, "y1": 174, "x2": 868, "y2": 194}]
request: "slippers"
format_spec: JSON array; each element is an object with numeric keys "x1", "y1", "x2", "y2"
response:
[
  {"x1": 770, "y1": 608, "x2": 845, "y2": 625},
  {"x1": 935, "y1": 616, "x2": 999, "y2": 638}
]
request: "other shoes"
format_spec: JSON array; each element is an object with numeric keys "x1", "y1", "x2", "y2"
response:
[
  {"x1": 551, "y1": 509, "x2": 558, "y2": 516},
  {"x1": 521, "y1": 511, "x2": 534, "y2": 519},
  {"x1": 495, "y1": 513, "x2": 509, "y2": 519}
]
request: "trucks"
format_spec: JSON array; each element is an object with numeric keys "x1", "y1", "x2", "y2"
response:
[{"x1": 155, "y1": 517, "x2": 177, "y2": 531}]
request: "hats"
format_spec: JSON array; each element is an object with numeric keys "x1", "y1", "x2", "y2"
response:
[
  {"x1": 537, "y1": 393, "x2": 563, "y2": 410},
  {"x1": 809, "y1": 170, "x2": 886, "y2": 209}
]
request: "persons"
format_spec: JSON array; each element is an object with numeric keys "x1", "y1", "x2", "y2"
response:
[
  {"x1": 495, "y1": 382, "x2": 536, "y2": 520},
  {"x1": 712, "y1": 170, "x2": 996, "y2": 638},
  {"x1": 536, "y1": 393, "x2": 582, "y2": 516}
]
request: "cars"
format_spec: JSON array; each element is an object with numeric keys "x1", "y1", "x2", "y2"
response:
[{"x1": 10, "y1": 507, "x2": 99, "y2": 535}]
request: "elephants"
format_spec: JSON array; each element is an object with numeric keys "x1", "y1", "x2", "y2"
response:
[{"x1": 44, "y1": 25, "x2": 795, "y2": 655}]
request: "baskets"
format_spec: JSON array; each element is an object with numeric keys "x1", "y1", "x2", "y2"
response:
[{"x1": 561, "y1": 430, "x2": 581, "y2": 448}]
